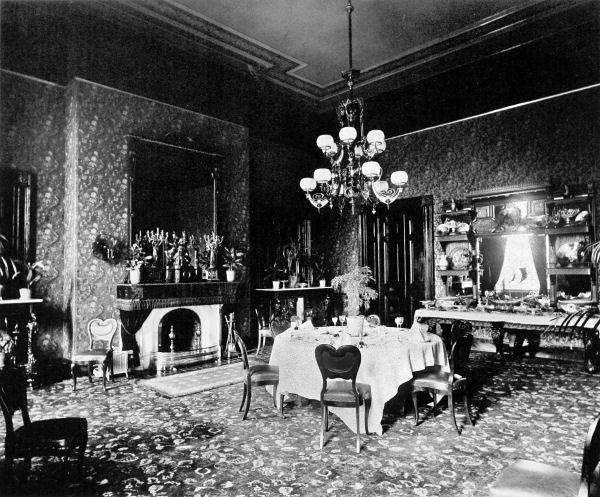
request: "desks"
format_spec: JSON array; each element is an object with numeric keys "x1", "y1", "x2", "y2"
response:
[
  {"x1": 255, "y1": 287, "x2": 334, "y2": 328},
  {"x1": 413, "y1": 306, "x2": 599, "y2": 372}
]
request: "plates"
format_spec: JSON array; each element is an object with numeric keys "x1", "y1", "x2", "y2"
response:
[
  {"x1": 556, "y1": 243, "x2": 587, "y2": 268},
  {"x1": 446, "y1": 246, "x2": 473, "y2": 271}
]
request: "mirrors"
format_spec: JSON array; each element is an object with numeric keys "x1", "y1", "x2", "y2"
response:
[{"x1": 123, "y1": 131, "x2": 223, "y2": 270}]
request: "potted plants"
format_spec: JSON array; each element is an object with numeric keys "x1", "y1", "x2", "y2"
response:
[{"x1": 270, "y1": 240, "x2": 318, "y2": 288}]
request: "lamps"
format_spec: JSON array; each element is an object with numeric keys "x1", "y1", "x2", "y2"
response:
[{"x1": 300, "y1": 2, "x2": 410, "y2": 215}]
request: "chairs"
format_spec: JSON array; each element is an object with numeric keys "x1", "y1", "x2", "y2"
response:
[
  {"x1": 411, "y1": 333, "x2": 475, "y2": 437},
  {"x1": 233, "y1": 330, "x2": 287, "y2": 420},
  {"x1": 0, "y1": 365, "x2": 87, "y2": 493},
  {"x1": 254, "y1": 305, "x2": 289, "y2": 357},
  {"x1": 315, "y1": 344, "x2": 373, "y2": 454},
  {"x1": 71, "y1": 317, "x2": 117, "y2": 393},
  {"x1": 490, "y1": 412, "x2": 600, "y2": 496}
]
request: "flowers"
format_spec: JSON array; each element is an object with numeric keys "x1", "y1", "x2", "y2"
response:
[
  {"x1": 222, "y1": 247, "x2": 243, "y2": 270},
  {"x1": 125, "y1": 245, "x2": 148, "y2": 270}
]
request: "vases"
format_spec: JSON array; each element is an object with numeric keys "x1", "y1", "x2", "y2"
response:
[
  {"x1": 226, "y1": 270, "x2": 234, "y2": 282},
  {"x1": 129, "y1": 270, "x2": 140, "y2": 284}
]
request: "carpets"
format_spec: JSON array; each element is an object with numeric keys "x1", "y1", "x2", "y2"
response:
[{"x1": 131, "y1": 358, "x2": 269, "y2": 398}]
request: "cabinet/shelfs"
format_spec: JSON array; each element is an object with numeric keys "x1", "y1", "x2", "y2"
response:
[
  {"x1": 431, "y1": 203, "x2": 481, "y2": 305},
  {"x1": 546, "y1": 182, "x2": 599, "y2": 310}
]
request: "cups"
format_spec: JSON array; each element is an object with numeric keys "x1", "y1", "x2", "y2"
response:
[
  {"x1": 272, "y1": 280, "x2": 280, "y2": 289},
  {"x1": 319, "y1": 280, "x2": 326, "y2": 287}
]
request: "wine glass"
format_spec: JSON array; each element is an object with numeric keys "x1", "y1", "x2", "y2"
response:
[
  {"x1": 396, "y1": 317, "x2": 403, "y2": 330},
  {"x1": 395, "y1": 318, "x2": 400, "y2": 329},
  {"x1": 332, "y1": 317, "x2": 338, "y2": 326},
  {"x1": 338, "y1": 316, "x2": 346, "y2": 327}
]
